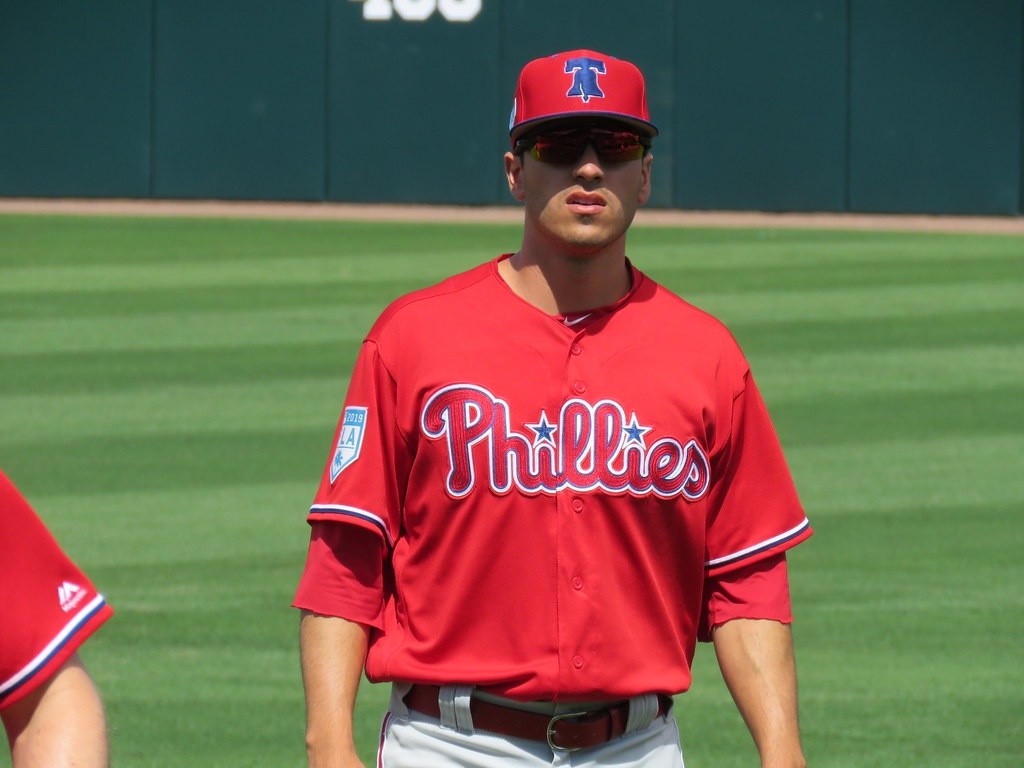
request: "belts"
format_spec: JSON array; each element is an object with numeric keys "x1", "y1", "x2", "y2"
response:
[{"x1": 403, "y1": 682, "x2": 677, "y2": 754}]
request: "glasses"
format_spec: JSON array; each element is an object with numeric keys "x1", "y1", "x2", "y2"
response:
[{"x1": 514, "y1": 129, "x2": 649, "y2": 162}]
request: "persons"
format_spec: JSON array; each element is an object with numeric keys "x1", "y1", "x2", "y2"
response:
[
  {"x1": 0, "y1": 470, "x2": 116, "y2": 768},
  {"x1": 291, "y1": 49, "x2": 808, "y2": 768}
]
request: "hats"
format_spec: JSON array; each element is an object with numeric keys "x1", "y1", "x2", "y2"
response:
[{"x1": 508, "y1": 48, "x2": 662, "y2": 145}]
